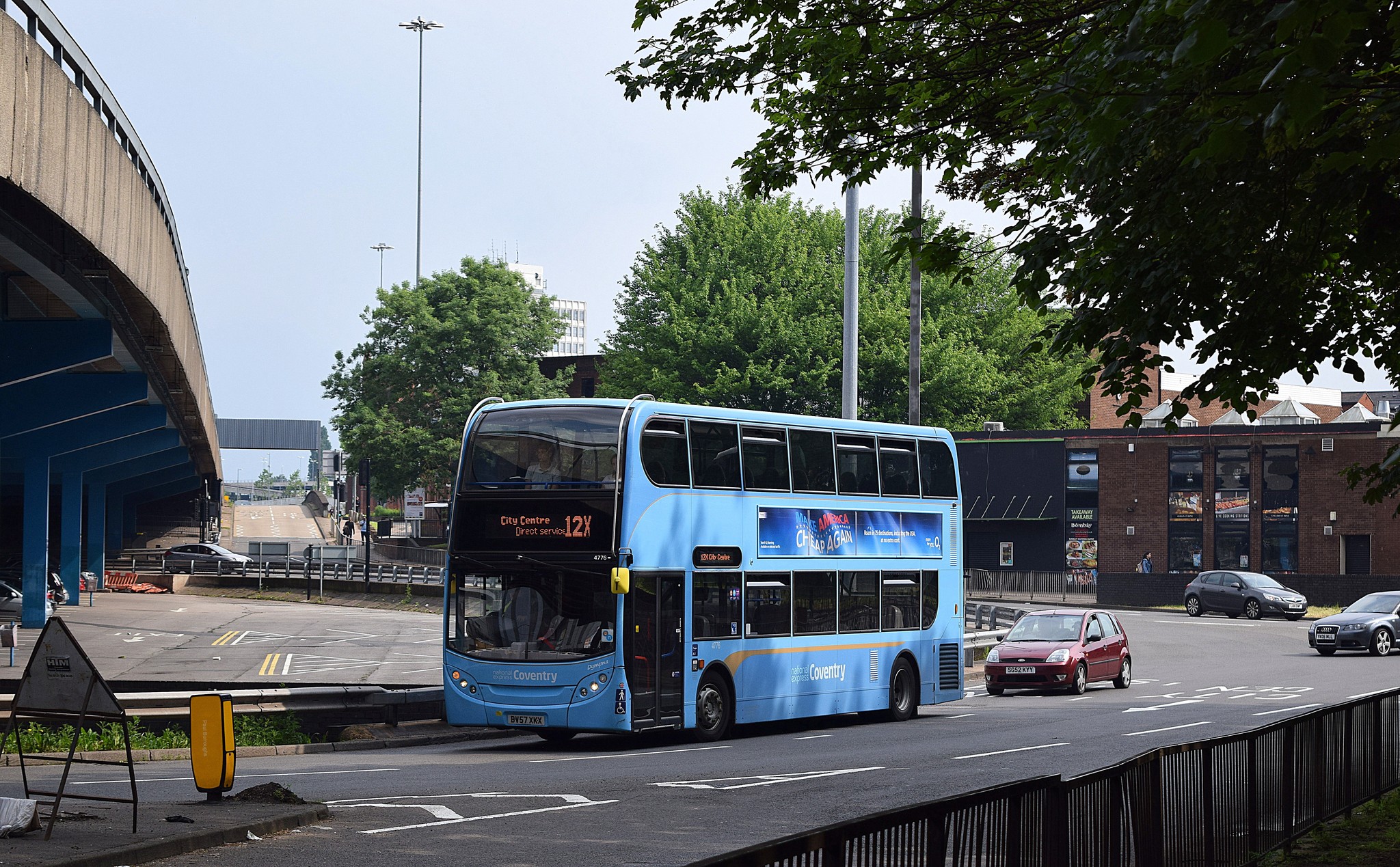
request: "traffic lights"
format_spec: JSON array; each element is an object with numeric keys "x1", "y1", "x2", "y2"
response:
[{"x1": 358, "y1": 460, "x2": 369, "y2": 486}]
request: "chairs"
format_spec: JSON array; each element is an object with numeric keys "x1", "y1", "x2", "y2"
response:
[
  {"x1": 694, "y1": 605, "x2": 934, "y2": 640},
  {"x1": 649, "y1": 461, "x2": 951, "y2": 497}
]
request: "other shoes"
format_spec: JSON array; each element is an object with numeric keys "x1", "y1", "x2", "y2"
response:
[{"x1": 362, "y1": 543, "x2": 364, "y2": 546}]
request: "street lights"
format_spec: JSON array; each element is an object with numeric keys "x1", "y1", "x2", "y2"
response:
[
  {"x1": 370, "y1": 241, "x2": 396, "y2": 388},
  {"x1": 400, "y1": 15, "x2": 442, "y2": 292}
]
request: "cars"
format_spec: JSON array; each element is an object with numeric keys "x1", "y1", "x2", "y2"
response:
[
  {"x1": 162, "y1": 543, "x2": 255, "y2": 575},
  {"x1": 1308, "y1": 591, "x2": 1400, "y2": 656},
  {"x1": 79, "y1": 569, "x2": 97, "y2": 593},
  {"x1": 0, "y1": 569, "x2": 66, "y2": 620},
  {"x1": 1184, "y1": 571, "x2": 1307, "y2": 620},
  {"x1": 985, "y1": 609, "x2": 1133, "y2": 695}
]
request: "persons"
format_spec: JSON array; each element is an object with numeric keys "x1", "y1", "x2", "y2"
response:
[
  {"x1": 360, "y1": 516, "x2": 367, "y2": 546},
  {"x1": 343, "y1": 517, "x2": 355, "y2": 546},
  {"x1": 499, "y1": 585, "x2": 530, "y2": 621},
  {"x1": 524, "y1": 443, "x2": 562, "y2": 490},
  {"x1": 330, "y1": 507, "x2": 334, "y2": 517},
  {"x1": 337, "y1": 504, "x2": 343, "y2": 524},
  {"x1": 598, "y1": 453, "x2": 623, "y2": 489},
  {"x1": 352, "y1": 496, "x2": 361, "y2": 513},
  {"x1": 1025, "y1": 618, "x2": 1081, "y2": 638},
  {"x1": 1142, "y1": 552, "x2": 1153, "y2": 573}
]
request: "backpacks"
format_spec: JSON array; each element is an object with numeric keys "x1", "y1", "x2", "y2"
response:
[{"x1": 1137, "y1": 559, "x2": 1146, "y2": 573}]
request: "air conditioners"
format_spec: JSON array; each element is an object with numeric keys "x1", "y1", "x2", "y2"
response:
[{"x1": 983, "y1": 422, "x2": 1003, "y2": 431}]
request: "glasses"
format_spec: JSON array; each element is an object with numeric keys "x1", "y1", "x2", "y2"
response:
[{"x1": 536, "y1": 451, "x2": 545, "y2": 454}]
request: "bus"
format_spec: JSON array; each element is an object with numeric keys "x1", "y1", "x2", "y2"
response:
[{"x1": 442, "y1": 393, "x2": 964, "y2": 745}]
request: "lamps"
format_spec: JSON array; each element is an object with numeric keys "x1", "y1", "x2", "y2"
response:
[
  {"x1": 1127, "y1": 508, "x2": 1135, "y2": 512},
  {"x1": 1204, "y1": 507, "x2": 1209, "y2": 510},
  {"x1": 1252, "y1": 507, "x2": 1257, "y2": 510}
]
